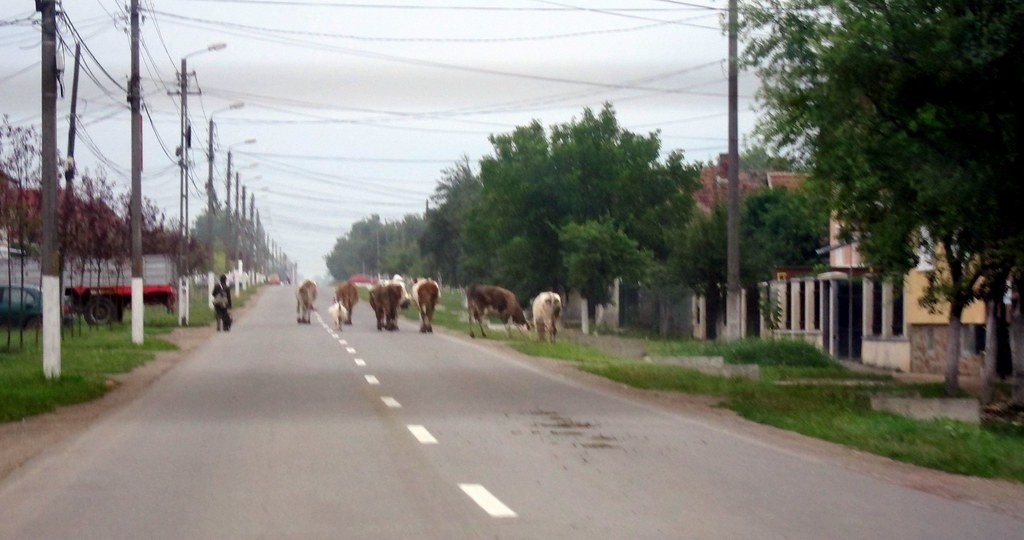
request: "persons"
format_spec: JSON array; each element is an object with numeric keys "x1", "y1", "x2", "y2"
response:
[{"x1": 212, "y1": 275, "x2": 232, "y2": 330}]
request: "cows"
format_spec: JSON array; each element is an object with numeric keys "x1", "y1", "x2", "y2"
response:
[
  {"x1": 369, "y1": 283, "x2": 403, "y2": 331},
  {"x1": 531, "y1": 291, "x2": 561, "y2": 344},
  {"x1": 466, "y1": 284, "x2": 532, "y2": 339},
  {"x1": 411, "y1": 277, "x2": 441, "y2": 333},
  {"x1": 328, "y1": 281, "x2": 358, "y2": 331},
  {"x1": 294, "y1": 279, "x2": 318, "y2": 324}
]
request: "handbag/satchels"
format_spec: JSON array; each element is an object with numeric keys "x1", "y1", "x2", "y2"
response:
[{"x1": 213, "y1": 294, "x2": 229, "y2": 308}]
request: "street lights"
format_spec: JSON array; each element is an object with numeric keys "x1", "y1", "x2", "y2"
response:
[
  {"x1": 205, "y1": 99, "x2": 245, "y2": 308},
  {"x1": 177, "y1": 43, "x2": 227, "y2": 324},
  {"x1": 225, "y1": 138, "x2": 257, "y2": 294}
]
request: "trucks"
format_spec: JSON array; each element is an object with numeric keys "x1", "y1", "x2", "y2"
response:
[{"x1": 64, "y1": 253, "x2": 177, "y2": 326}]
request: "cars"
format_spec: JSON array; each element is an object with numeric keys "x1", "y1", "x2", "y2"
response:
[{"x1": 0, "y1": 282, "x2": 74, "y2": 326}]
